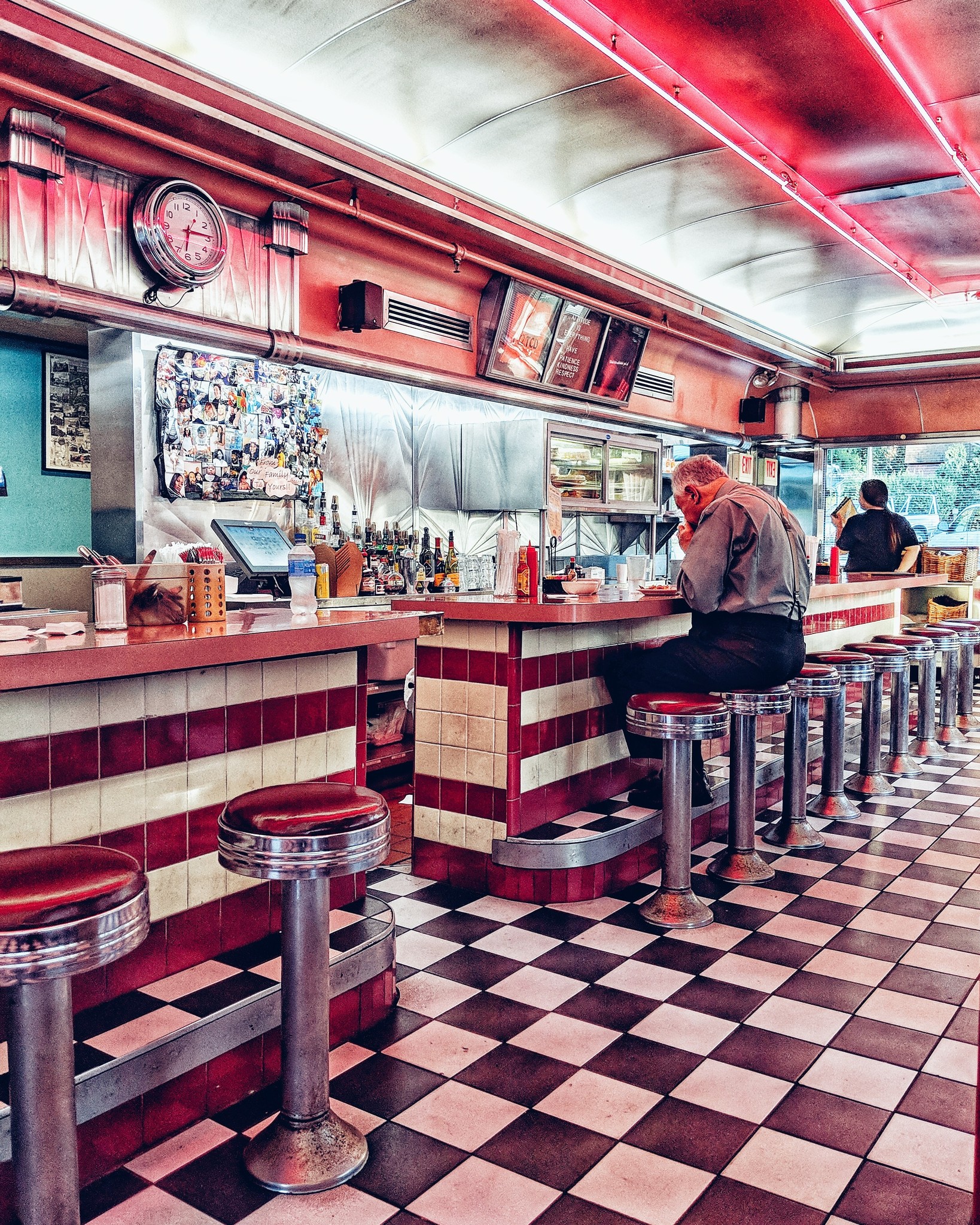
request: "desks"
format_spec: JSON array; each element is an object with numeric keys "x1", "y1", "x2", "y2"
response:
[{"x1": 924, "y1": 581, "x2": 973, "y2": 618}]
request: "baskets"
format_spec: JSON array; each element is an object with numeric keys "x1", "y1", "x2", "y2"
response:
[
  {"x1": 927, "y1": 598, "x2": 968, "y2": 622},
  {"x1": 908, "y1": 547, "x2": 936, "y2": 573},
  {"x1": 923, "y1": 549, "x2": 979, "y2": 582}
]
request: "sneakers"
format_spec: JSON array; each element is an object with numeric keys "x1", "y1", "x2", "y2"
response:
[{"x1": 628, "y1": 766, "x2": 715, "y2": 808}]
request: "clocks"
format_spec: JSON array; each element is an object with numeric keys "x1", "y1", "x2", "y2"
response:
[{"x1": 132, "y1": 178, "x2": 229, "y2": 289}]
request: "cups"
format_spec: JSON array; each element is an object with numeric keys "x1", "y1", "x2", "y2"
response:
[
  {"x1": 456, "y1": 553, "x2": 494, "y2": 593},
  {"x1": 615, "y1": 564, "x2": 628, "y2": 581}
]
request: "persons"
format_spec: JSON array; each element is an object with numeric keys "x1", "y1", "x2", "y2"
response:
[
  {"x1": 157, "y1": 347, "x2": 327, "y2": 499},
  {"x1": 601, "y1": 453, "x2": 811, "y2": 809},
  {"x1": 836, "y1": 479, "x2": 920, "y2": 572}
]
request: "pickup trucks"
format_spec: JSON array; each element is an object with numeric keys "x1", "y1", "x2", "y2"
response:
[{"x1": 824, "y1": 494, "x2": 941, "y2": 545}]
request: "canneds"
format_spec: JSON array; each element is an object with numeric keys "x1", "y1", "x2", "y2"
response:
[
  {"x1": 315, "y1": 563, "x2": 330, "y2": 598},
  {"x1": 317, "y1": 610, "x2": 330, "y2": 618}
]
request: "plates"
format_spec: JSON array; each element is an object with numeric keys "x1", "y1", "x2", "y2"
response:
[{"x1": 636, "y1": 588, "x2": 678, "y2": 596}]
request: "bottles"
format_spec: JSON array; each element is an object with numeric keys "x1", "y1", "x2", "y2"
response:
[
  {"x1": 517, "y1": 546, "x2": 530, "y2": 598},
  {"x1": 567, "y1": 557, "x2": 577, "y2": 582},
  {"x1": 288, "y1": 533, "x2": 318, "y2": 615},
  {"x1": 830, "y1": 545, "x2": 840, "y2": 576},
  {"x1": 526, "y1": 540, "x2": 538, "y2": 597},
  {"x1": 90, "y1": 568, "x2": 128, "y2": 631},
  {"x1": 296, "y1": 490, "x2": 459, "y2": 597}
]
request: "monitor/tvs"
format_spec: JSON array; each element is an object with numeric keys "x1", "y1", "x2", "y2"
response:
[{"x1": 210, "y1": 519, "x2": 294, "y2": 579}]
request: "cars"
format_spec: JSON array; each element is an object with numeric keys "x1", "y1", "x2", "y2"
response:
[{"x1": 927, "y1": 503, "x2": 980, "y2": 557}]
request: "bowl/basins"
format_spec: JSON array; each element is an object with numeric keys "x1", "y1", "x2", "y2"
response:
[
  {"x1": 561, "y1": 581, "x2": 599, "y2": 595},
  {"x1": 577, "y1": 578, "x2": 602, "y2": 592}
]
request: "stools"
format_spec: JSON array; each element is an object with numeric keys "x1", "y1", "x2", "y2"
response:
[
  {"x1": 219, "y1": 782, "x2": 392, "y2": 1192},
  {"x1": 624, "y1": 618, "x2": 980, "y2": 929},
  {"x1": 0, "y1": 845, "x2": 151, "y2": 1225}
]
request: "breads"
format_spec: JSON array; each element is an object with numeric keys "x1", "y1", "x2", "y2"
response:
[
  {"x1": 608, "y1": 457, "x2": 637, "y2": 465},
  {"x1": 549, "y1": 446, "x2": 601, "y2": 498}
]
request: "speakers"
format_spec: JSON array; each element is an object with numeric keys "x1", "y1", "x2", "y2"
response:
[
  {"x1": 341, "y1": 280, "x2": 382, "y2": 330},
  {"x1": 740, "y1": 398, "x2": 765, "y2": 423}
]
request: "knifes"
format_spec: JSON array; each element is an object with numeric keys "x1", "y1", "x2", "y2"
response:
[{"x1": 126, "y1": 549, "x2": 157, "y2": 613}]
